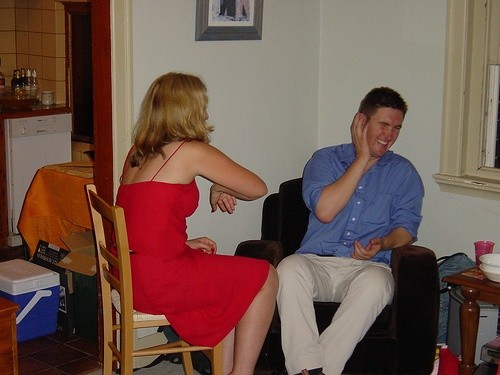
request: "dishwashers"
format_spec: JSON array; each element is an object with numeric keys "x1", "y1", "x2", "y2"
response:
[{"x1": 3, "y1": 113, "x2": 73, "y2": 248}]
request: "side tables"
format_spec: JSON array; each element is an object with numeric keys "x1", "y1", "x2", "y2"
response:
[{"x1": 441, "y1": 267, "x2": 500, "y2": 375}]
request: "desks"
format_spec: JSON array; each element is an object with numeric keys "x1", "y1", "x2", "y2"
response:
[
  {"x1": 0, "y1": 297, "x2": 20, "y2": 375},
  {"x1": 18, "y1": 159, "x2": 99, "y2": 296}
]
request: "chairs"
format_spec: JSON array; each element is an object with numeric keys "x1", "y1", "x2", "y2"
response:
[{"x1": 82, "y1": 182, "x2": 226, "y2": 375}]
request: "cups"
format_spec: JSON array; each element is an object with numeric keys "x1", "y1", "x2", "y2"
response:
[
  {"x1": 41, "y1": 90, "x2": 53, "y2": 106},
  {"x1": 474, "y1": 241, "x2": 495, "y2": 270}
]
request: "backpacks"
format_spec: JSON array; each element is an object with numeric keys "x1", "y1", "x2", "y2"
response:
[{"x1": 436, "y1": 253, "x2": 475, "y2": 343}]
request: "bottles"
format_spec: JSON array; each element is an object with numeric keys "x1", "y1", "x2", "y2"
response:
[
  {"x1": 0, "y1": 58, "x2": 6, "y2": 89},
  {"x1": 438, "y1": 345, "x2": 460, "y2": 375},
  {"x1": 11, "y1": 67, "x2": 39, "y2": 105}
]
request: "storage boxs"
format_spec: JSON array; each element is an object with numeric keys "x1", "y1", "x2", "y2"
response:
[
  {"x1": 0, "y1": 257, "x2": 62, "y2": 344},
  {"x1": 71, "y1": 271, "x2": 99, "y2": 344}
]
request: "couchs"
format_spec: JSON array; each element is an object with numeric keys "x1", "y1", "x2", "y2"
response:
[{"x1": 236, "y1": 176, "x2": 444, "y2": 375}]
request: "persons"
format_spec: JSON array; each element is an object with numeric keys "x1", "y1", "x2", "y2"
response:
[
  {"x1": 116, "y1": 72, "x2": 279, "y2": 375},
  {"x1": 275, "y1": 87, "x2": 425, "y2": 375}
]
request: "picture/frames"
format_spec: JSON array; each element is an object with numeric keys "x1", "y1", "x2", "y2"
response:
[{"x1": 194, "y1": 0, "x2": 264, "y2": 41}]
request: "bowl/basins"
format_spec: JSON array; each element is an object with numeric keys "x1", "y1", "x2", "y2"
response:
[{"x1": 479, "y1": 253, "x2": 500, "y2": 282}]
